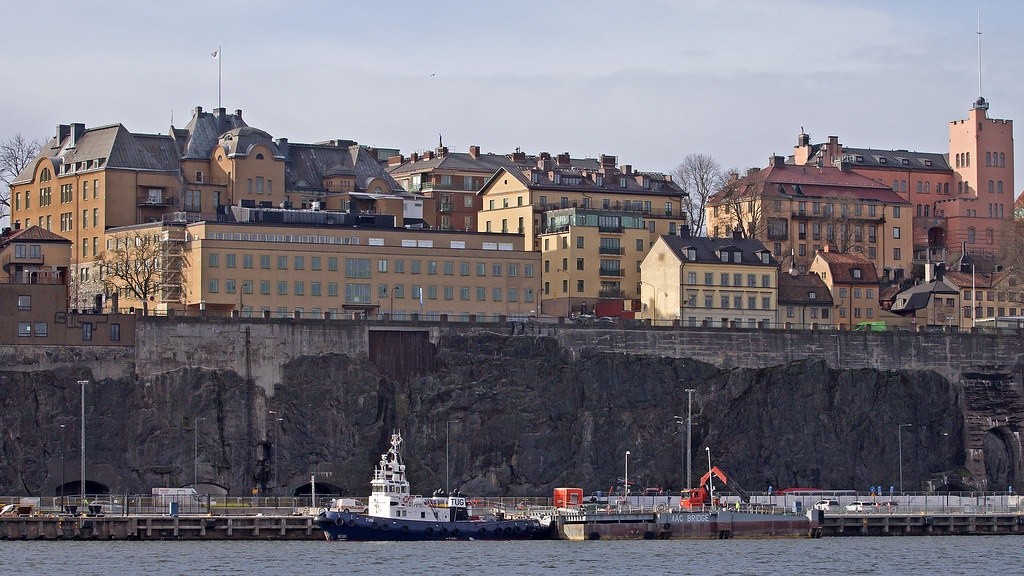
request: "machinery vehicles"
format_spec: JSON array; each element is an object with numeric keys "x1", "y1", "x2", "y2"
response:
[{"x1": 681, "y1": 464, "x2": 753, "y2": 507}]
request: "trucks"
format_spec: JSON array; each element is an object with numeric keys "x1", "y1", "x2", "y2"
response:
[
  {"x1": 853, "y1": 321, "x2": 886, "y2": 331},
  {"x1": 152, "y1": 488, "x2": 203, "y2": 509}
]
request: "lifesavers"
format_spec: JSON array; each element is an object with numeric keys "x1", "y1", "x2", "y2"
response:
[
  {"x1": 400, "y1": 524, "x2": 409, "y2": 533},
  {"x1": 381, "y1": 523, "x2": 391, "y2": 531},
  {"x1": 479, "y1": 528, "x2": 487, "y2": 535},
  {"x1": 514, "y1": 526, "x2": 522, "y2": 534},
  {"x1": 664, "y1": 523, "x2": 670, "y2": 529},
  {"x1": 505, "y1": 526, "x2": 513, "y2": 534},
  {"x1": 335, "y1": 517, "x2": 344, "y2": 526},
  {"x1": 453, "y1": 528, "x2": 460, "y2": 536},
  {"x1": 426, "y1": 527, "x2": 434, "y2": 535},
  {"x1": 440, "y1": 528, "x2": 448, "y2": 536},
  {"x1": 496, "y1": 527, "x2": 504, "y2": 535},
  {"x1": 348, "y1": 519, "x2": 356, "y2": 527}
]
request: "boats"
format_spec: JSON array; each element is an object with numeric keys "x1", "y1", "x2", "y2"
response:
[
  {"x1": 312, "y1": 427, "x2": 560, "y2": 542},
  {"x1": 556, "y1": 501, "x2": 811, "y2": 540}
]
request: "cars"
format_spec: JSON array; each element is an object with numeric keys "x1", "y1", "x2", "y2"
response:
[
  {"x1": 575, "y1": 314, "x2": 596, "y2": 323},
  {"x1": 599, "y1": 316, "x2": 617, "y2": 323},
  {"x1": 813, "y1": 499, "x2": 840, "y2": 511},
  {"x1": 843, "y1": 500, "x2": 872, "y2": 512}
]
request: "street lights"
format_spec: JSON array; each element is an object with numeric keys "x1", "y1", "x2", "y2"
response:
[
  {"x1": 77, "y1": 379, "x2": 91, "y2": 508},
  {"x1": 267, "y1": 409, "x2": 277, "y2": 489},
  {"x1": 239, "y1": 284, "x2": 247, "y2": 317},
  {"x1": 685, "y1": 389, "x2": 696, "y2": 489},
  {"x1": 537, "y1": 288, "x2": 545, "y2": 320},
  {"x1": 194, "y1": 416, "x2": 205, "y2": 491},
  {"x1": 557, "y1": 268, "x2": 572, "y2": 319},
  {"x1": 636, "y1": 280, "x2": 668, "y2": 326},
  {"x1": 446, "y1": 420, "x2": 459, "y2": 494},
  {"x1": 625, "y1": 451, "x2": 631, "y2": 498},
  {"x1": 898, "y1": 423, "x2": 913, "y2": 495},
  {"x1": 705, "y1": 446, "x2": 715, "y2": 506},
  {"x1": 391, "y1": 286, "x2": 399, "y2": 320}
]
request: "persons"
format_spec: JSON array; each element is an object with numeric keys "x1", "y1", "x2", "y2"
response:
[{"x1": 713, "y1": 495, "x2": 741, "y2": 514}]
